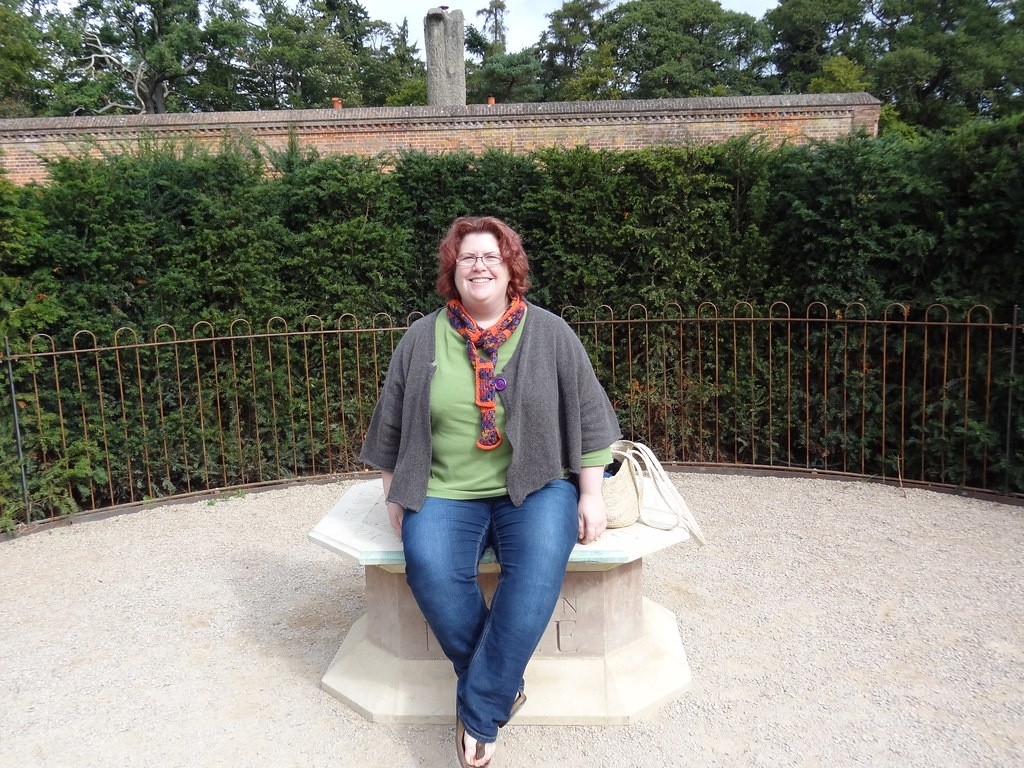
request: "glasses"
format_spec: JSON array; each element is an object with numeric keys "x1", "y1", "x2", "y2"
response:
[{"x1": 455, "y1": 252, "x2": 504, "y2": 266}]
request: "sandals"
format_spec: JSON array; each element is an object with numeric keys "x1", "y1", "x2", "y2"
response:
[
  {"x1": 456, "y1": 710, "x2": 495, "y2": 768},
  {"x1": 499, "y1": 677, "x2": 526, "y2": 723}
]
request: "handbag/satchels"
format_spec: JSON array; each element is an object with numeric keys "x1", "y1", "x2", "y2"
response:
[{"x1": 601, "y1": 439, "x2": 707, "y2": 545}]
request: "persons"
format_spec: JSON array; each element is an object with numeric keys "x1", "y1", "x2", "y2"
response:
[{"x1": 360, "y1": 216, "x2": 620, "y2": 768}]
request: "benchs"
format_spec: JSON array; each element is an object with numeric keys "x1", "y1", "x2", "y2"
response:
[{"x1": 308, "y1": 478, "x2": 695, "y2": 729}]
